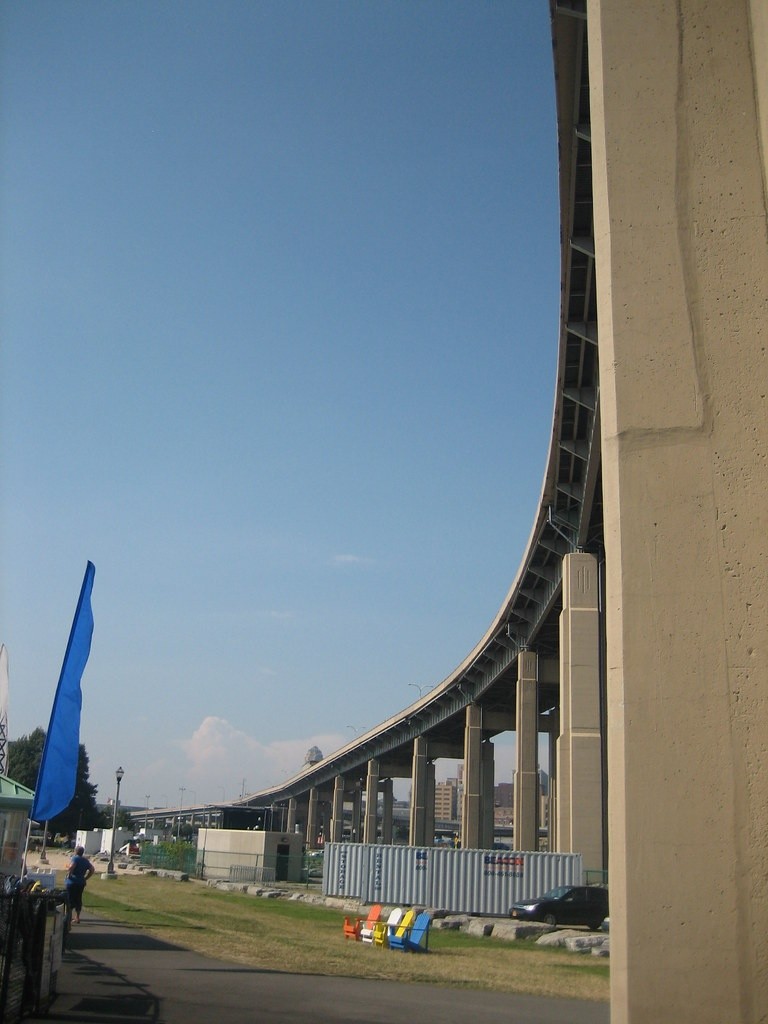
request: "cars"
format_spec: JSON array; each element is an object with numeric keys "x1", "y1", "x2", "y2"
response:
[{"x1": 510, "y1": 884, "x2": 608, "y2": 930}]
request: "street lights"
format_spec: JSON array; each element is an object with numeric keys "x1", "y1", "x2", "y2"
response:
[
  {"x1": 144, "y1": 794, "x2": 150, "y2": 838},
  {"x1": 100, "y1": 767, "x2": 124, "y2": 880},
  {"x1": 177, "y1": 785, "x2": 188, "y2": 837}
]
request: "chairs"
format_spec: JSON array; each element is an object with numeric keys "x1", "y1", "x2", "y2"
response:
[{"x1": 343, "y1": 905, "x2": 431, "y2": 953}]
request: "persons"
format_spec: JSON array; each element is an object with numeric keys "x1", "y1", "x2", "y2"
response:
[{"x1": 64, "y1": 846, "x2": 94, "y2": 924}]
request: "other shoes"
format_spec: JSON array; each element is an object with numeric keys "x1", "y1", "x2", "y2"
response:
[{"x1": 72, "y1": 919, "x2": 80, "y2": 923}]
